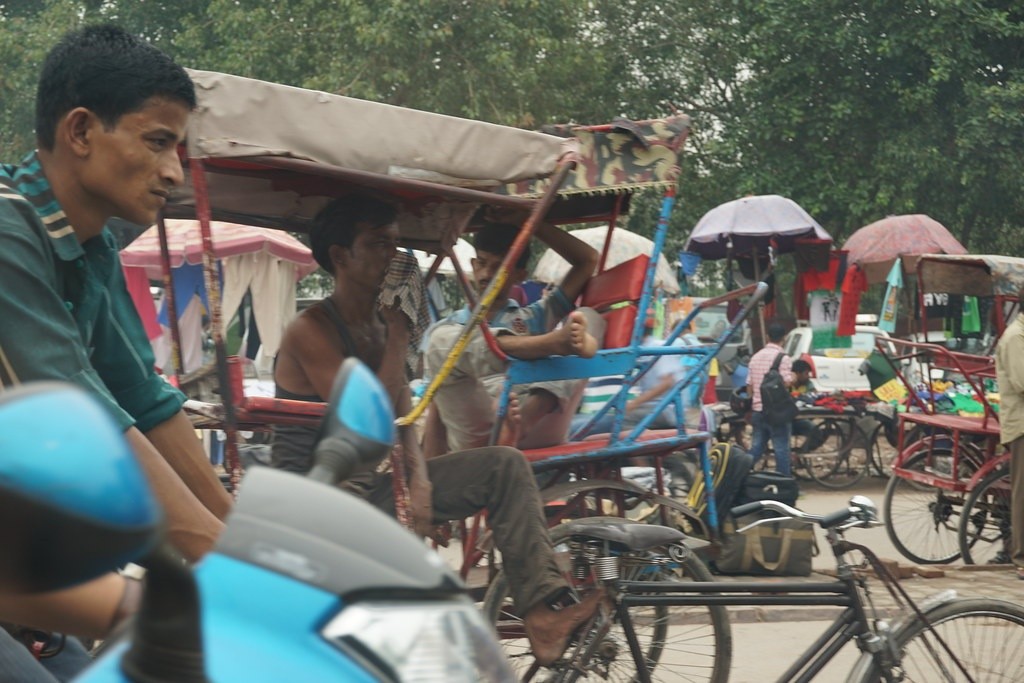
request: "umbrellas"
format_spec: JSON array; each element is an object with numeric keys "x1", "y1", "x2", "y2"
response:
[
  {"x1": 840, "y1": 213, "x2": 972, "y2": 343},
  {"x1": 681, "y1": 191, "x2": 835, "y2": 345},
  {"x1": 878, "y1": 259, "x2": 905, "y2": 335}
]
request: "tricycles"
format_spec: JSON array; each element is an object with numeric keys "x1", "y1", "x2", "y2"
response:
[{"x1": 136, "y1": 64, "x2": 1024, "y2": 683}]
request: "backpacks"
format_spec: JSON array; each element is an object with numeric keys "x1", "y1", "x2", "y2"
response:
[{"x1": 760, "y1": 354, "x2": 799, "y2": 426}]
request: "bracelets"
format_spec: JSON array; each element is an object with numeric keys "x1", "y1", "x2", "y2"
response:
[{"x1": 109, "y1": 562, "x2": 146, "y2": 631}]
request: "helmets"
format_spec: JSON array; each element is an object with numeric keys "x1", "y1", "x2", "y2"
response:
[{"x1": 729, "y1": 386, "x2": 753, "y2": 414}]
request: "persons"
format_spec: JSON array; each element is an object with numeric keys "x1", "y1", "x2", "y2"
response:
[
  {"x1": 568, "y1": 314, "x2": 826, "y2": 480},
  {"x1": 0, "y1": 23, "x2": 265, "y2": 683},
  {"x1": 271, "y1": 193, "x2": 607, "y2": 669},
  {"x1": 991, "y1": 292, "x2": 1024, "y2": 580},
  {"x1": 417, "y1": 200, "x2": 607, "y2": 551}
]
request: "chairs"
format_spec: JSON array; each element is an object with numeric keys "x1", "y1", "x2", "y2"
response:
[
  {"x1": 182, "y1": 358, "x2": 412, "y2": 526},
  {"x1": 519, "y1": 254, "x2": 652, "y2": 464},
  {"x1": 897, "y1": 346, "x2": 1003, "y2": 433}
]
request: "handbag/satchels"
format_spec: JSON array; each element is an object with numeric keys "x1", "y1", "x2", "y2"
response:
[{"x1": 686, "y1": 438, "x2": 819, "y2": 575}]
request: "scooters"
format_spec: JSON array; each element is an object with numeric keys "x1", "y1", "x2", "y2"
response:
[{"x1": 0, "y1": 356, "x2": 521, "y2": 680}]
